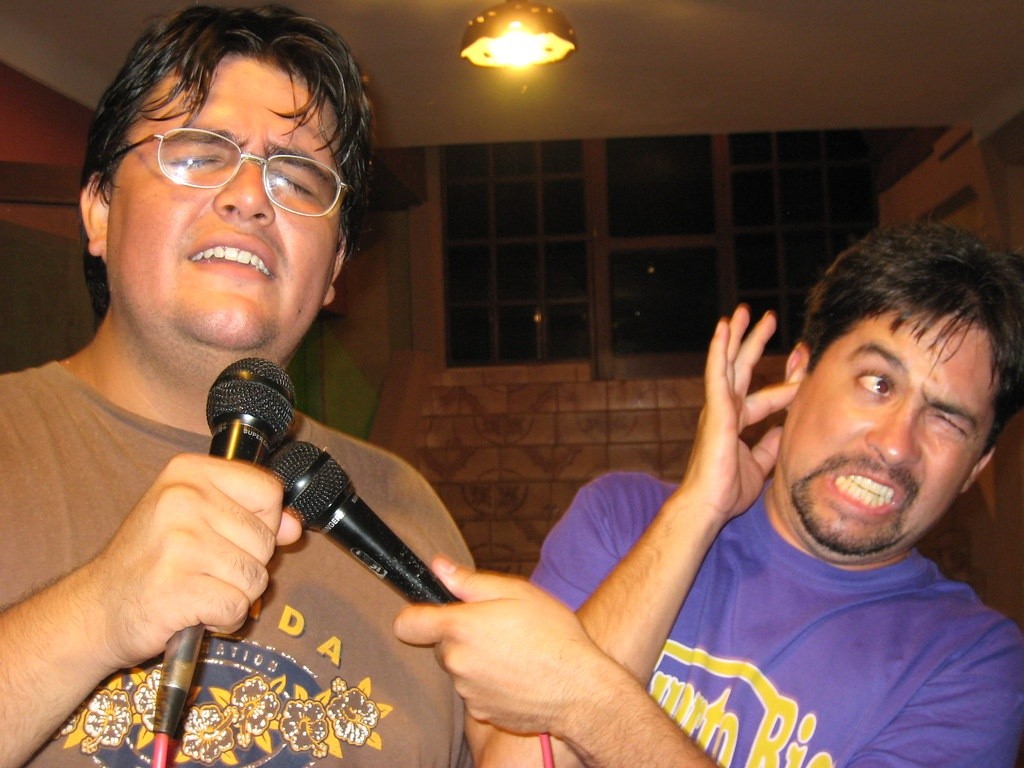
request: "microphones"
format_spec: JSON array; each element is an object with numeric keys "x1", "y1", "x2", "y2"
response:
[
  {"x1": 262, "y1": 439, "x2": 460, "y2": 609},
  {"x1": 154, "y1": 357, "x2": 298, "y2": 737}
]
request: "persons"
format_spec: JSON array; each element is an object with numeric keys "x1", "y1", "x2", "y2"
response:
[
  {"x1": 0, "y1": 0, "x2": 477, "y2": 768},
  {"x1": 395, "y1": 220, "x2": 1024, "y2": 768}
]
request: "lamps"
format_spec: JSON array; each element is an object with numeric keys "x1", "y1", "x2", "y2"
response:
[{"x1": 458, "y1": 0, "x2": 580, "y2": 69}]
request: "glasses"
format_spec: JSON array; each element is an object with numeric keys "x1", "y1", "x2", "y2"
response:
[{"x1": 114, "y1": 128, "x2": 350, "y2": 218}]
